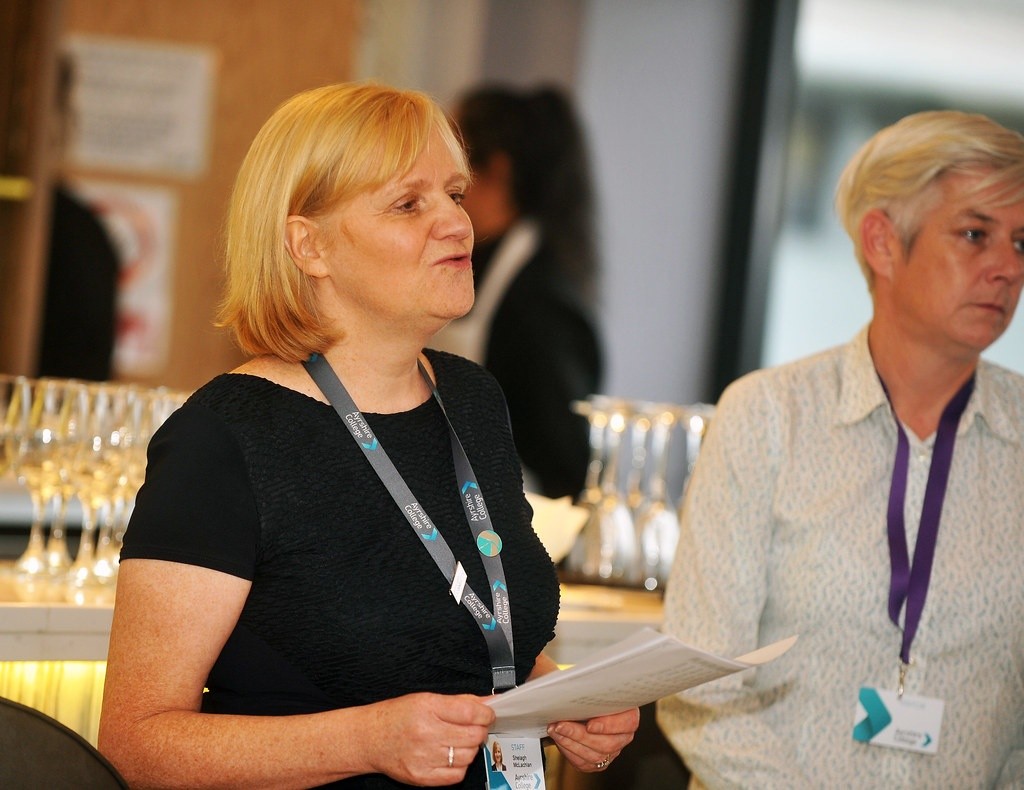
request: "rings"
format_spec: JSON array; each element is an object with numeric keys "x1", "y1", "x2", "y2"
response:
[
  {"x1": 448, "y1": 747, "x2": 454, "y2": 766},
  {"x1": 595, "y1": 756, "x2": 610, "y2": 768}
]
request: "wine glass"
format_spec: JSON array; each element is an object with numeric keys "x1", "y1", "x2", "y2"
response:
[
  {"x1": 566, "y1": 396, "x2": 718, "y2": 592},
  {"x1": 0, "y1": 375, "x2": 199, "y2": 604}
]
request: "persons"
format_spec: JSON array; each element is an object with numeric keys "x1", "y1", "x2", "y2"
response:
[
  {"x1": 26, "y1": 58, "x2": 122, "y2": 383},
  {"x1": 655, "y1": 104, "x2": 1023, "y2": 790},
  {"x1": 429, "y1": 80, "x2": 605, "y2": 505},
  {"x1": 491, "y1": 741, "x2": 506, "y2": 771},
  {"x1": 96, "y1": 82, "x2": 639, "y2": 790}
]
